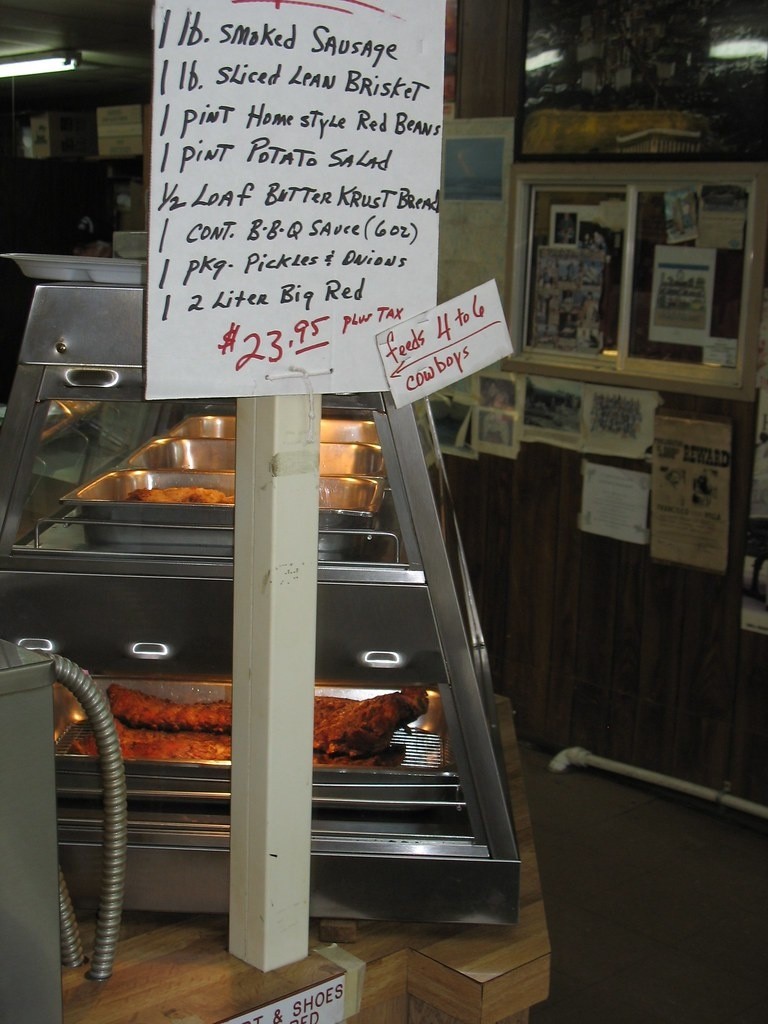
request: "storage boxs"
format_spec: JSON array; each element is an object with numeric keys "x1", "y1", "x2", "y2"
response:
[{"x1": 30, "y1": 104, "x2": 144, "y2": 159}]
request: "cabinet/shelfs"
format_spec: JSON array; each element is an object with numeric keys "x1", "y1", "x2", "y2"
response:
[{"x1": 0, "y1": 285, "x2": 522, "y2": 928}]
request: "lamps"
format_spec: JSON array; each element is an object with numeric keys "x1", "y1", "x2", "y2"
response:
[{"x1": 0, "y1": 50, "x2": 83, "y2": 78}]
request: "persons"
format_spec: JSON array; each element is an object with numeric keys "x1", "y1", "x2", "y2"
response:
[
  {"x1": 533, "y1": 248, "x2": 603, "y2": 350},
  {"x1": 556, "y1": 212, "x2": 576, "y2": 243},
  {"x1": 482, "y1": 391, "x2": 509, "y2": 444}
]
